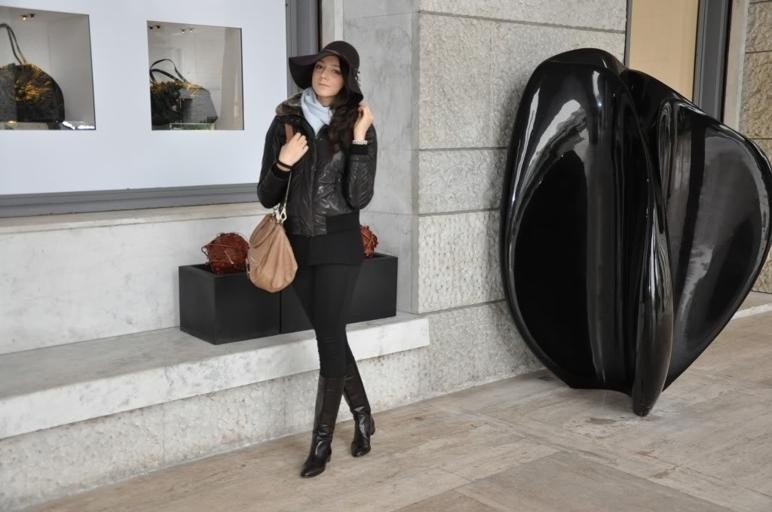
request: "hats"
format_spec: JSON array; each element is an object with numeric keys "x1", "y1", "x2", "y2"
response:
[{"x1": 289, "y1": 41, "x2": 363, "y2": 102}]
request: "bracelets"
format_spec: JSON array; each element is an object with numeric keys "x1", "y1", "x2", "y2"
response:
[{"x1": 276, "y1": 159, "x2": 294, "y2": 169}]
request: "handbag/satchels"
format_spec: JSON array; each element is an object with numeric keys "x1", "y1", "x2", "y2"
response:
[
  {"x1": 0, "y1": 63, "x2": 65, "y2": 123},
  {"x1": 149, "y1": 58, "x2": 218, "y2": 125},
  {"x1": 245, "y1": 213, "x2": 299, "y2": 295}
]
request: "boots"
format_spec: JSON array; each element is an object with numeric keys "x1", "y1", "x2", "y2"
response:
[{"x1": 300, "y1": 372, "x2": 376, "y2": 479}]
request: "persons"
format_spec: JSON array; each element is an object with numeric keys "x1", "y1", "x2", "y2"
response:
[{"x1": 255, "y1": 40, "x2": 378, "y2": 480}]
